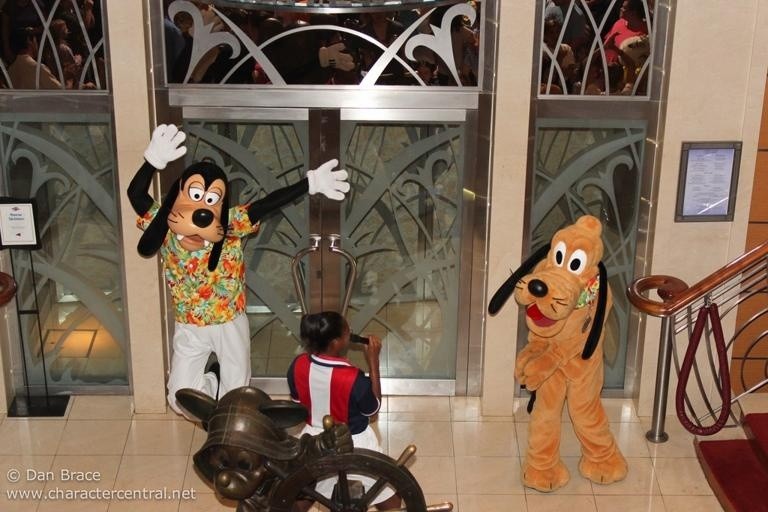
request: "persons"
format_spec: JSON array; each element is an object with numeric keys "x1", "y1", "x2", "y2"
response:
[
  {"x1": 287, "y1": 312, "x2": 401, "y2": 512},
  {"x1": 6, "y1": 0, "x2": 654, "y2": 95}
]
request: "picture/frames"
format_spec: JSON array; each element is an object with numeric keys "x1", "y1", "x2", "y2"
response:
[{"x1": 673, "y1": 139, "x2": 741, "y2": 224}]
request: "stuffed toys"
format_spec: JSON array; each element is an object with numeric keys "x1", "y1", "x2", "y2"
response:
[
  {"x1": 127, "y1": 124, "x2": 350, "y2": 431},
  {"x1": 489, "y1": 215, "x2": 628, "y2": 492}
]
